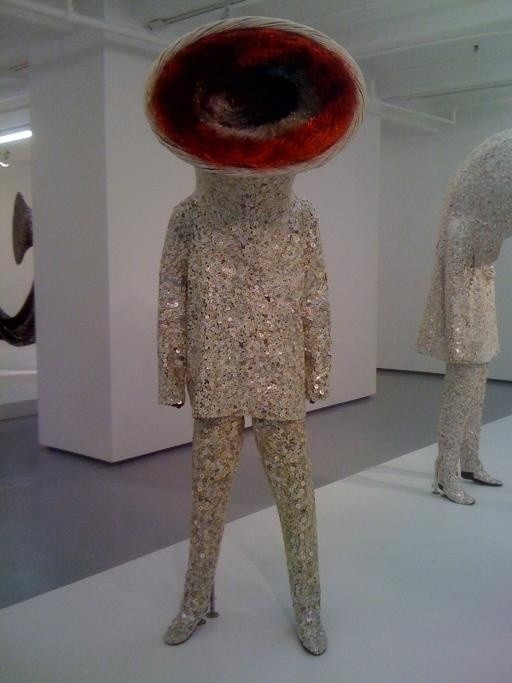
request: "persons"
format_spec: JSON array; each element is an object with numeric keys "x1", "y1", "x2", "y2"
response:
[
  {"x1": 156, "y1": 193, "x2": 331, "y2": 655},
  {"x1": 413, "y1": 214, "x2": 502, "y2": 505}
]
